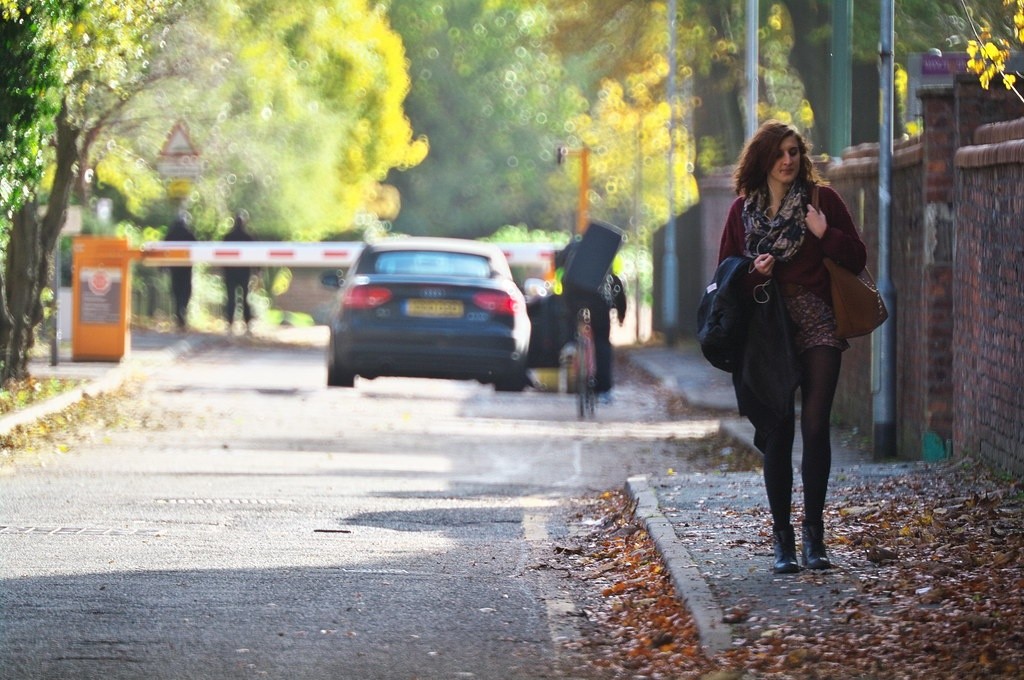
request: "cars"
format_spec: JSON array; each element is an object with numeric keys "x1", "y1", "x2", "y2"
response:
[
  {"x1": 488, "y1": 239, "x2": 577, "y2": 369},
  {"x1": 319, "y1": 235, "x2": 533, "y2": 393}
]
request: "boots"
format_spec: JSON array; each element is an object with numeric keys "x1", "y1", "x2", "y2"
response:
[
  {"x1": 802, "y1": 519, "x2": 830, "y2": 569},
  {"x1": 773, "y1": 524, "x2": 800, "y2": 574}
]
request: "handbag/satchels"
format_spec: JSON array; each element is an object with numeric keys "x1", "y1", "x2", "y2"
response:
[{"x1": 811, "y1": 186, "x2": 889, "y2": 339}]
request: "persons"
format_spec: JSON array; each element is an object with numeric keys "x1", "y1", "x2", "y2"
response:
[
  {"x1": 719, "y1": 122, "x2": 867, "y2": 573},
  {"x1": 222, "y1": 218, "x2": 260, "y2": 326},
  {"x1": 159, "y1": 220, "x2": 197, "y2": 328},
  {"x1": 557, "y1": 240, "x2": 626, "y2": 401}
]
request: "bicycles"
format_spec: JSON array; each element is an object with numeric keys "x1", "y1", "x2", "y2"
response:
[{"x1": 560, "y1": 305, "x2": 610, "y2": 419}]
